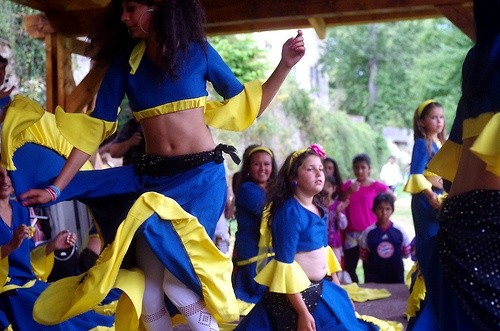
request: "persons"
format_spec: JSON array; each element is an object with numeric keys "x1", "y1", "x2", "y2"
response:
[
  {"x1": 0, "y1": 0, "x2": 500, "y2": 331},
  {"x1": 232, "y1": 143, "x2": 405, "y2": 331}
]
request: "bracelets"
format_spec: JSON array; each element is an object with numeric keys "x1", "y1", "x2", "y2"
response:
[
  {"x1": 48, "y1": 188, "x2": 57, "y2": 200},
  {"x1": 45, "y1": 188, "x2": 54, "y2": 201},
  {"x1": 49, "y1": 185, "x2": 61, "y2": 197}
]
